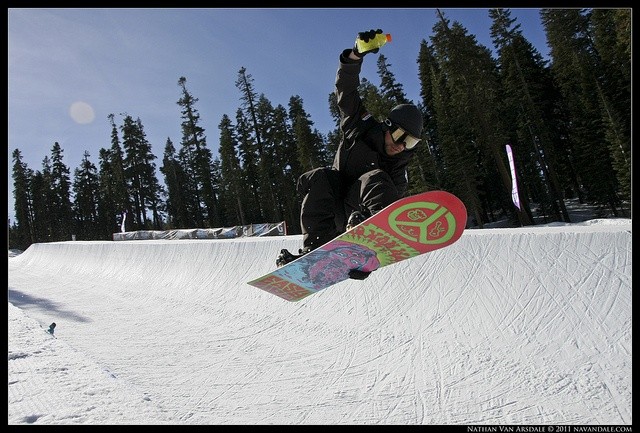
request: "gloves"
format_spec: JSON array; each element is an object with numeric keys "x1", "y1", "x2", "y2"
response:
[
  {"x1": 353, "y1": 29, "x2": 383, "y2": 60},
  {"x1": 348, "y1": 269, "x2": 370, "y2": 281}
]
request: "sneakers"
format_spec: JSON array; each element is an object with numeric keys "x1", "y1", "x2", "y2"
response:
[
  {"x1": 276, "y1": 247, "x2": 318, "y2": 269},
  {"x1": 346, "y1": 211, "x2": 368, "y2": 232}
]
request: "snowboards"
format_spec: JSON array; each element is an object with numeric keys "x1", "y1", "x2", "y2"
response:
[{"x1": 247, "y1": 189, "x2": 467, "y2": 302}]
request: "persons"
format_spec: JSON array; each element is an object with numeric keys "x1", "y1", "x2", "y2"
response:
[{"x1": 275, "y1": 27, "x2": 424, "y2": 281}]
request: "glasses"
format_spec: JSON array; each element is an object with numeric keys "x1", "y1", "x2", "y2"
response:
[{"x1": 385, "y1": 118, "x2": 423, "y2": 152}]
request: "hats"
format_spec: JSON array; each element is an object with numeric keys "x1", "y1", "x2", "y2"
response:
[{"x1": 385, "y1": 105, "x2": 425, "y2": 141}]
